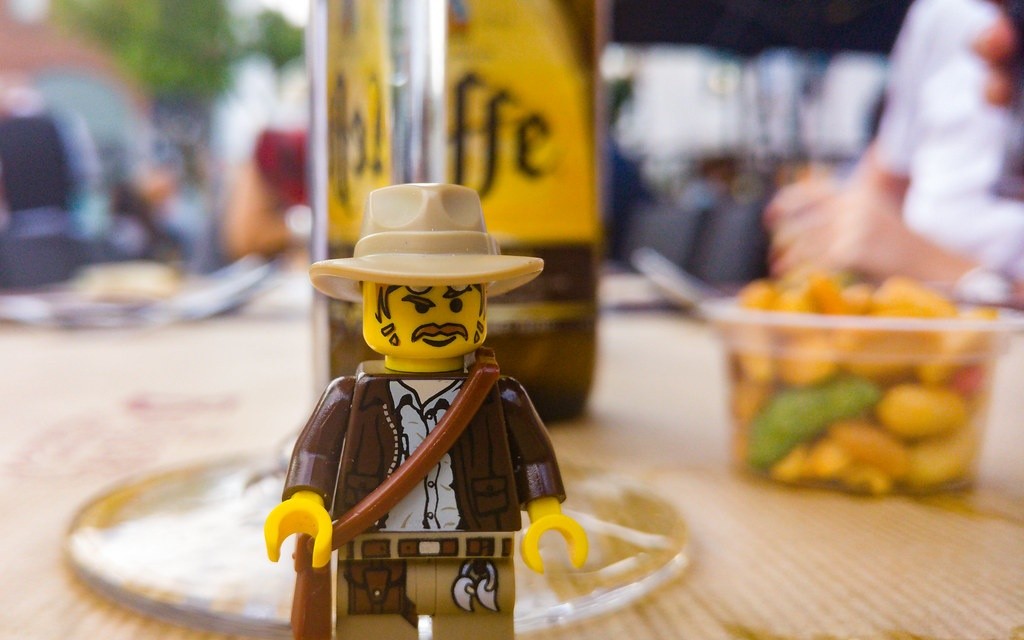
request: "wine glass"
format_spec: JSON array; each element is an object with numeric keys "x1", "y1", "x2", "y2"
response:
[{"x1": 69, "y1": 0, "x2": 695, "y2": 634}]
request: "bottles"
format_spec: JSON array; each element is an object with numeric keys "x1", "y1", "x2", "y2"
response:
[{"x1": 309, "y1": 0, "x2": 604, "y2": 420}]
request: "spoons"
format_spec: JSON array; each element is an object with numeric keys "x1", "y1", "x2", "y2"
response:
[{"x1": 0, "y1": 254, "x2": 284, "y2": 333}]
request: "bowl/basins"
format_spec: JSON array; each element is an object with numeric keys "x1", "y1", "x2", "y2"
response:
[{"x1": 701, "y1": 299, "x2": 1024, "y2": 501}]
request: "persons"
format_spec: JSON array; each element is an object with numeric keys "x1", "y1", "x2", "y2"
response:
[
  {"x1": 595, "y1": 0, "x2": 1024, "y2": 307},
  {"x1": 265, "y1": 183, "x2": 587, "y2": 640}
]
